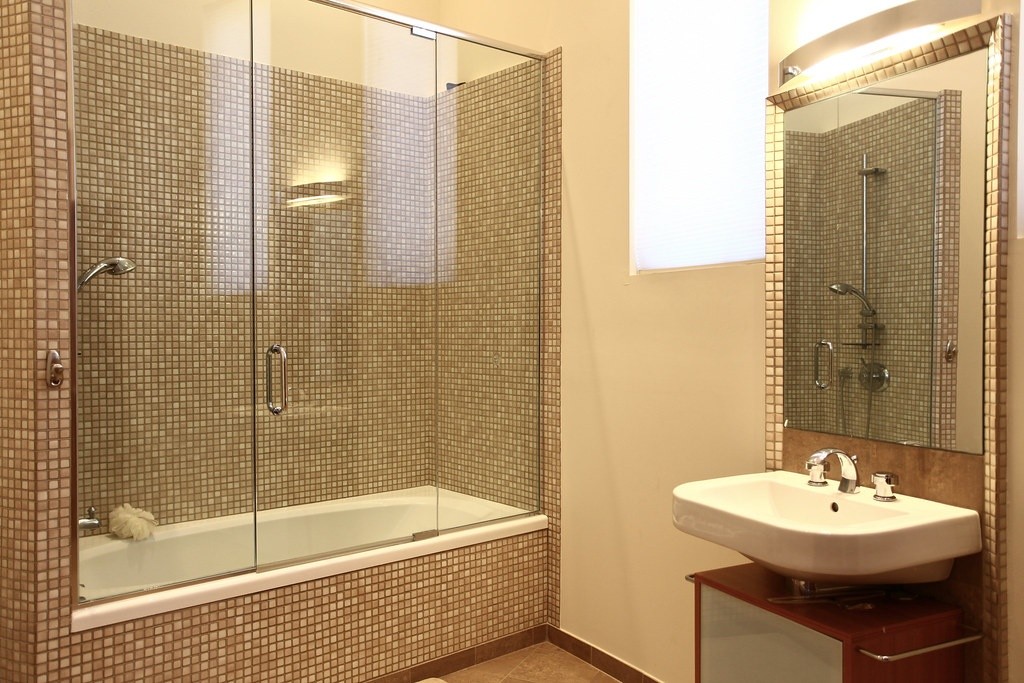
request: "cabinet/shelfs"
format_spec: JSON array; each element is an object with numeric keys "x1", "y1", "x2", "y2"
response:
[{"x1": 686, "y1": 563, "x2": 984, "y2": 683}]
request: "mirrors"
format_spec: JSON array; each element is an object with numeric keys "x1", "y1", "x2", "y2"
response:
[{"x1": 782, "y1": 46, "x2": 987, "y2": 454}]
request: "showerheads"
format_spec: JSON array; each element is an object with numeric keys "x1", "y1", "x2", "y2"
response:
[
  {"x1": 76, "y1": 255, "x2": 139, "y2": 294},
  {"x1": 827, "y1": 281, "x2": 877, "y2": 318}
]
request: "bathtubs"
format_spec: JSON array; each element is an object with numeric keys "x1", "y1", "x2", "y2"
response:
[{"x1": 74, "y1": 482, "x2": 546, "y2": 682}]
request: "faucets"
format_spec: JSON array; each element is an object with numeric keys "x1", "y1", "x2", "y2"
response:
[
  {"x1": 802, "y1": 445, "x2": 860, "y2": 495},
  {"x1": 78, "y1": 505, "x2": 102, "y2": 530}
]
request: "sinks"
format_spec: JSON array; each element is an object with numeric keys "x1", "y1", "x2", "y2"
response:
[{"x1": 668, "y1": 467, "x2": 985, "y2": 586}]
